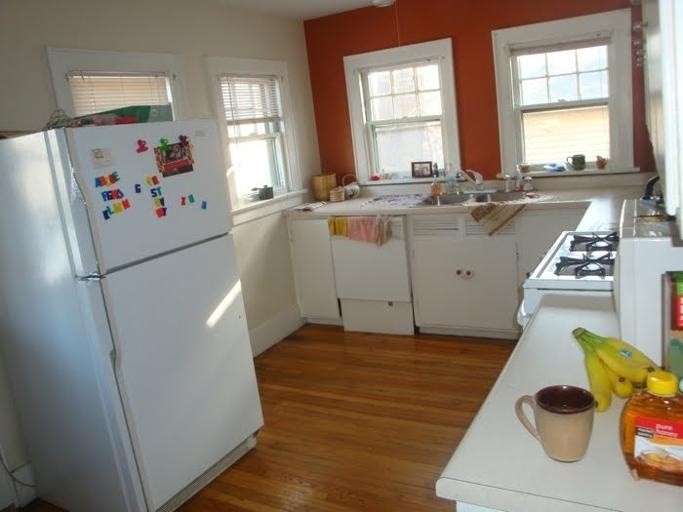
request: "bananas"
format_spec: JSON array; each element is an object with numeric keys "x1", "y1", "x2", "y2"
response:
[{"x1": 571, "y1": 327, "x2": 664, "y2": 412}]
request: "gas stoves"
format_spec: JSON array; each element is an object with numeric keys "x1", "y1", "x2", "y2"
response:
[{"x1": 523, "y1": 230, "x2": 618, "y2": 291}]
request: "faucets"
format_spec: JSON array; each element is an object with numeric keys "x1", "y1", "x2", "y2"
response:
[
  {"x1": 495, "y1": 174, "x2": 512, "y2": 192},
  {"x1": 459, "y1": 169, "x2": 483, "y2": 191}
]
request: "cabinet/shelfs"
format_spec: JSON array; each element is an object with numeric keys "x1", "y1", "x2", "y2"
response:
[
  {"x1": 286, "y1": 215, "x2": 341, "y2": 326},
  {"x1": 407, "y1": 213, "x2": 519, "y2": 341},
  {"x1": 517, "y1": 209, "x2": 584, "y2": 301},
  {"x1": 631, "y1": 0, "x2": 676, "y2": 217},
  {"x1": 328, "y1": 216, "x2": 415, "y2": 337}
]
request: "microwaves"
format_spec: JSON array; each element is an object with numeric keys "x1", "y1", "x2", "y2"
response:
[{"x1": 611, "y1": 196, "x2": 682, "y2": 373}]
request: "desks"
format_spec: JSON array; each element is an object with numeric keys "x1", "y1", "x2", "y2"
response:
[{"x1": 433, "y1": 294, "x2": 681, "y2": 511}]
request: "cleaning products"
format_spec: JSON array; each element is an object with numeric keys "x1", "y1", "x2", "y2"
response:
[
  {"x1": 513, "y1": 163, "x2": 525, "y2": 192},
  {"x1": 443, "y1": 163, "x2": 457, "y2": 194},
  {"x1": 431, "y1": 175, "x2": 441, "y2": 195}
]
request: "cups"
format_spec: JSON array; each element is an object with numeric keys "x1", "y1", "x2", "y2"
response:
[
  {"x1": 516, "y1": 386, "x2": 597, "y2": 464},
  {"x1": 596, "y1": 156, "x2": 608, "y2": 170},
  {"x1": 312, "y1": 173, "x2": 344, "y2": 202},
  {"x1": 567, "y1": 154, "x2": 586, "y2": 170}
]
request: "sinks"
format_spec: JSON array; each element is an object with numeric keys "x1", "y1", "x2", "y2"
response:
[
  {"x1": 476, "y1": 190, "x2": 530, "y2": 203},
  {"x1": 415, "y1": 193, "x2": 476, "y2": 206}
]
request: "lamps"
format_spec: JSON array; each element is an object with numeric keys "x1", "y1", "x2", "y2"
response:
[{"x1": 371, "y1": 0, "x2": 395, "y2": 8}]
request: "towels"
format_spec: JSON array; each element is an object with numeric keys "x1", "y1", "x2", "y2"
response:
[
  {"x1": 460, "y1": 201, "x2": 529, "y2": 237},
  {"x1": 326, "y1": 215, "x2": 392, "y2": 247}
]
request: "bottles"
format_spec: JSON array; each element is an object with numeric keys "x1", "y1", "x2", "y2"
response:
[
  {"x1": 514, "y1": 174, "x2": 533, "y2": 192},
  {"x1": 430, "y1": 163, "x2": 461, "y2": 197},
  {"x1": 619, "y1": 371, "x2": 682, "y2": 487}
]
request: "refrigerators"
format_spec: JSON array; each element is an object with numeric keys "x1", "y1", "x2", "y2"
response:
[{"x1": 0, "y1": 115, "x2": 264, "y2": 510}]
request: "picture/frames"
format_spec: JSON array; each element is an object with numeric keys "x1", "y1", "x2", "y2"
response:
[{"x1": 411, "y1": 161, "x2": 432, "y2": 178}]
